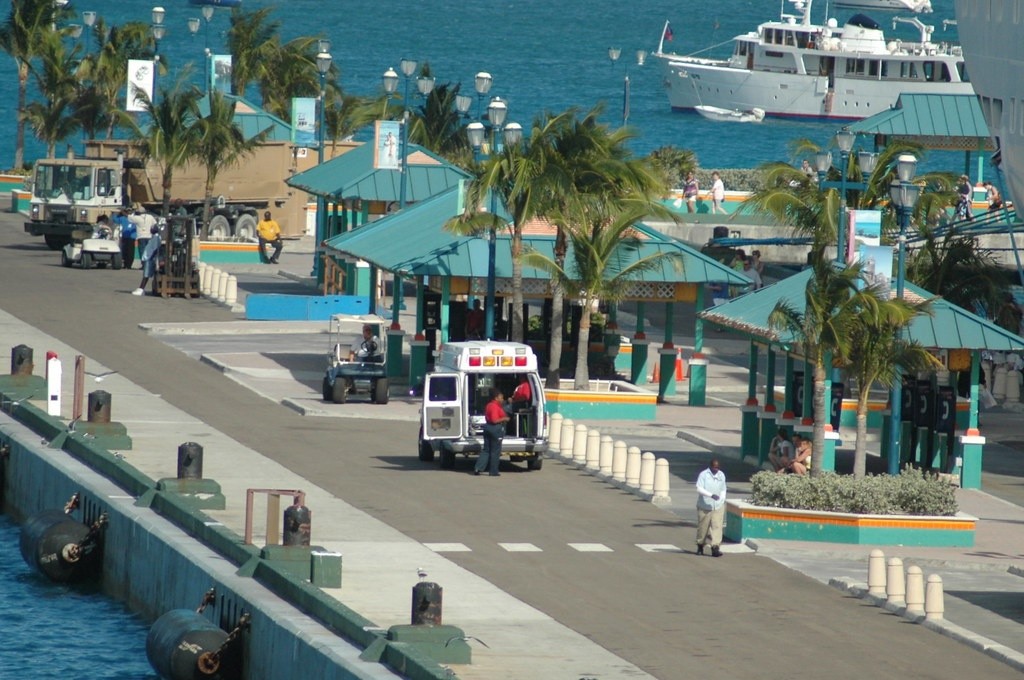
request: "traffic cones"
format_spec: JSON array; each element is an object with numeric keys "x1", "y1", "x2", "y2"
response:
[
  {"x1": 676, "y1": 346, "x2": 686, "y2": 381},
  {"x1": 650, "y1": 362, "x2": 660, "y2": 383},
  {"x1": 683, "y1": 356, "x2": 693, "y2": 378}
]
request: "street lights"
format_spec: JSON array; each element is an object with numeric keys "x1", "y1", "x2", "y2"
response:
[
  {"x1": 68, "y1": 10, "x2": 97, "y2": 157},
  {"x1": 186, "y1": 4, "x2": 215, "y2": 93},
  {"x1": 151, "y1": 6, "x2": 167, "y2": 109},
  {"x1": 607, "y1": 44, "x2": 650, "y2": 152},
  {"x1": 466, "y1": 96, "x2": 524, "y2": 342},
  {"x1": 382, "y1": 53, "x2": 436, "y2": 310},
  {"x1": 886, "y1": 153, "x2": 921, "y2": 474},
  {"x1": 813, "y1": 123, "x2": 880, "y2": 385},
  {"x1": 310, "y1": 37, "x2": 333, "y2": 278}
]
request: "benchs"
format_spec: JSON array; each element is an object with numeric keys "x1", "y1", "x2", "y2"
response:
[
  {"x1": 334, "y1": 344, "x2": 356, "y2": 362},
  {"x1": 513, "y1": 412, "x2": 533, "y2": 438}
]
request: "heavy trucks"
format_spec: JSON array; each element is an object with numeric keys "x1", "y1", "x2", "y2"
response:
[{"x1": 22, "y1": 139, "x2": 295, "y2": 251}]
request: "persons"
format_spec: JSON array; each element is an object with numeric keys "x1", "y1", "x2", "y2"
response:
[
  {"x1": 767, "y1": 428, "x2": 812, "y2": 475},
  {"x1": 356, "y1": 326, "x2": 380, "y2": 357},
  {"x1": 695, "y1": 459, "x2": 727, "y2": 557},
  {"x1": 256, "y1": 211, "x2": 283, "y2": 264},
  {"x1": 170, "y1": 197, "x2": 188, "y2": 242},
  {"x1": 949, "y1": 175, "x2": 976, "y2": 223},
  {"x1": 798, "y1": 33, "x2": 808, "y2": 48},
  {"x1": 956, "y1": 359, "x2": 986, "y2": 428},
  {"x1": 131, "y1": 225, "x2": 161, "y2": 296},
  {"x1": 474, "y1": 390, "x2": 510, "y2": 476},
  {"x1": 807, "y1": 174, "x2": 817, "y2": 181},
  {"x1": 799, "y1": 158, "x2": 812, "y2": 172},
  {"x1": 703, "y1": 250, "x2": 765, "y2": 333},
  {"x1": 983, "y1": 181, "x2": 1001, "y2": 222},
  {"x1": 383, "y1": 132, "x2": 396, "y2": 164},
  {"x1": 681, "y1": 170, "x2": 700, "y2": 213},
  {"x1": 704, "y1": 171, "x2": 728, "y2": 215},
  {"x1": 501, "y1": 374, "x2": 531, "y2": 436},
  {"x1": 112, "y1": 207, "x2": 137, "y2": 269},
  {"x1": 127, "y1": 205, "x2": 157, "y2": 270},
  {"x1": 465, "y1": 298, "x2": 485, "y2": 340}
]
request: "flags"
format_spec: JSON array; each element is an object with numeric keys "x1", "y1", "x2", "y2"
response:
[{"x1": 665, "y1": 24, "x2": 674, "y2": 41}]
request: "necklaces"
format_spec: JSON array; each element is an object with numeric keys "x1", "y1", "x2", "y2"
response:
[{"x1": 139, "y1": 213, "x2": 147, "y2": 231}]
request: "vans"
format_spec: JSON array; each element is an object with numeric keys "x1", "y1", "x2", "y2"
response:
[{"x1": 418, "y1": 338, "x2": 550, "y2": 471}]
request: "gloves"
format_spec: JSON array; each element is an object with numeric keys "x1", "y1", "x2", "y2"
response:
[{"x1": 712, "y1": 495, "x2": 719, "y2": 500}]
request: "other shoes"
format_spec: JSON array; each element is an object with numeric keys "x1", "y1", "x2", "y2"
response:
[
  {"x1": 489, "y1": 473, "x2": 500, "y2": 476},
  {"x1": 711, "y1": 547, "x2": 723, "y2": 557},
  {"x1": 697, "y1": 545, "x2": 703, "y2": 554},
  {"x1": 474, "y1": 469, "x2": 479, "y2": 474}
]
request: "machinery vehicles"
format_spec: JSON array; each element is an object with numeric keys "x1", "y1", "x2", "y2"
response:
[{"x1": 152, "y1": 215, "x2": 201, "y2": 301}]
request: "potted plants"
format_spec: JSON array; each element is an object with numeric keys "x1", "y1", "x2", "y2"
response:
[{"x1": 528, "y1": 310, "x2": 605, "y2": 342}]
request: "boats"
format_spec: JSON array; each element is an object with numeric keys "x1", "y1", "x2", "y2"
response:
[
  {"x1": 694, "y1": 104, "x2": 766, "y2": 124},
  {"x1": 832, "y1": 0, "x2": 934, "y2": 14},
  {"x1": 650, "y1": 0, "x2": 975, "y2": 123}
]
